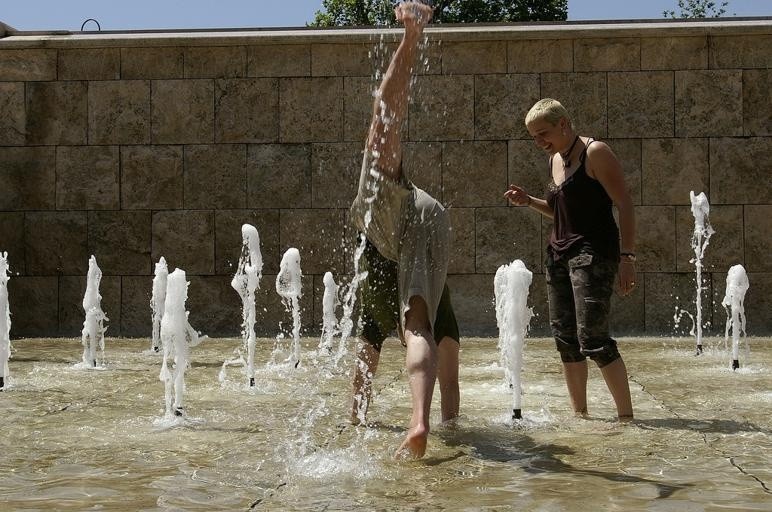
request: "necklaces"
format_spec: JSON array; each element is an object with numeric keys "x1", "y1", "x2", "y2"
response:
[{"x1": 560, "y1": 136, "x2": 579, "y2": 167}]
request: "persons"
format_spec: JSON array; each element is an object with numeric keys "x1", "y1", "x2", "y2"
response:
[
  {"x1": 344, "y1": 0, "x2": 461, "y2": 464},
  {"x1": 503, "y1": 96, "x2": 638, "y2": 420}
]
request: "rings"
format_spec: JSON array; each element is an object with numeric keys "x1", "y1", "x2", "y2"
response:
[{"x1": 630, "y1": 281, "x2": 635, "y2": 286}]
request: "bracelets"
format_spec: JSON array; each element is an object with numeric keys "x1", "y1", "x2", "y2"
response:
[{"x1": 619, "y1": 252, "x2": 637, "y2": 265}]
